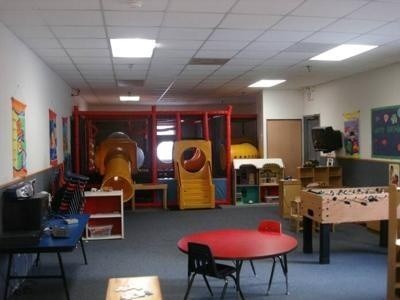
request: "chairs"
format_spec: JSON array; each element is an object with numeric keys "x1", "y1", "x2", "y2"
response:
[
  {"x1": 183, "y1": 241, "x2": 239, "y2": 300},
  {"x1": 236, "y1": 220, "x2": 282, "y2": 276}
]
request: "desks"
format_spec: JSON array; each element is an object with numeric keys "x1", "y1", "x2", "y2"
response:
[
  {"x1": 0, "y1": 213, "x2": 91, "y2": 300},
  {"x1": 177, "y1": 230, "x2": 298, "y2": 295}
]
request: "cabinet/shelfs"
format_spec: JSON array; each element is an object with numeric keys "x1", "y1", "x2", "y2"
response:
[
  {"x1": 232, "y1": 158, "x2": 284, "y2": 207},
  {"x1": 81, "y1": 188, "x2": 125, "y2": 241},
  {"x1": 297, "y1": 165, "x2": 343, "y2": 189}
]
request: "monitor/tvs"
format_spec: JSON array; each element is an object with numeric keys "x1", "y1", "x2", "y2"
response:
[{"x1": 311, "y1": 126, "x2": 342, "y2": 151}]
